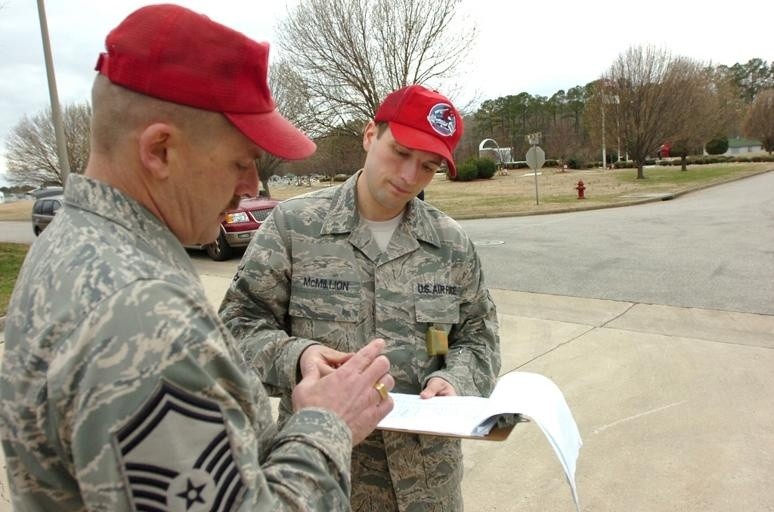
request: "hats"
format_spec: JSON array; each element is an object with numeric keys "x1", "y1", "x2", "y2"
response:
[
  {"x1": 374, "y1": 85, "x2": 462, "y2": 177},
  {"x1": 95, "y1": 4, "x2": 318, "y2": 160}
]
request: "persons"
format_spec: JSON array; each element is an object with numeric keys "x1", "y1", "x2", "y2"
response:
[
  {"x1": 217, "y1": 81, "x2": 502, "y2": 510},
  {"x1": 0, "y1": 2, "x2": 399, "y2": 510}
]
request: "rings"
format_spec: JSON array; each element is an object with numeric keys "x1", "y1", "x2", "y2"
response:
[{"x1": 376, "y1": 383, "x2": 390, "y2": 399}]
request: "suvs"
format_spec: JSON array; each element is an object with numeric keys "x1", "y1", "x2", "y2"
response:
[
  {"x1": 181, "y1": 190, "x2": 280, "y2": 261},
  {"x1": 26, "y1": 186, "x2": 68, "y2": 238}
]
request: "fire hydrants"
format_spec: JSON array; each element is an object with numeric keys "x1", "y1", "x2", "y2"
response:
[{"x1": 574, "y1": 180, "x2": 586, "y2": 200}]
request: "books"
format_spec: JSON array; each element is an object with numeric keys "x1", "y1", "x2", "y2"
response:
[{"x1": 377, "y1": 371, "x2": 588, "y2": 512}]
